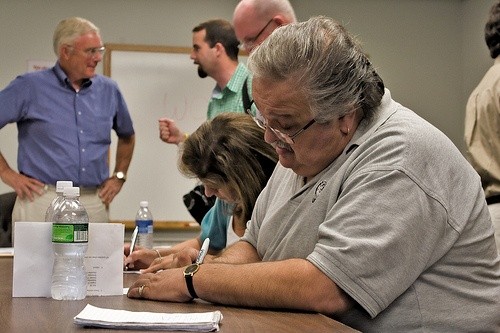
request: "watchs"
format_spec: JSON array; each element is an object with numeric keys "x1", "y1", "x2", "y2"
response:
[
  {"x1": 183, "y1": 263, "x2": 200, "y2": 299},
  {"x1": 114, "y1": 171, "x2": 126, "y2": 182}
]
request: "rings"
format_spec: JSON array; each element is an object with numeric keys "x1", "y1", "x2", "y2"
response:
[{"x1": 138, "y1": 285, "x2": 145, "y2": 297}]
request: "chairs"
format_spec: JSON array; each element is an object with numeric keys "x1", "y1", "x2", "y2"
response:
[{"x1": 486, "y1": 194, "x2": 500, "y2": 257}]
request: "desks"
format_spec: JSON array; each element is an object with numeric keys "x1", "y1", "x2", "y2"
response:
[{"x1": 0, "y1": 255, "x2": 364, "y2": 333}]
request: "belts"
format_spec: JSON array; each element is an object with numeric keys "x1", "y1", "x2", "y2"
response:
[{"x1": 486, "y1": 194, "x2": 500, "y2": 205}]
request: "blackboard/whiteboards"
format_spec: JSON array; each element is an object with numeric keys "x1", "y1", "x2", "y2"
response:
[{"x1": 102, "y1": 42, "x2": 251, "y2": 232}]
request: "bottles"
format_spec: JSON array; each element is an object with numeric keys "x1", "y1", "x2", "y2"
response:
[
  {"x1": 51, "y1": 187, "x2": 90, "y2": 301},
  {"x1": 135, "y1": 200, "x2": 154, "y2": 250},
  {"x1": 45, "y1": 181, "x2": 73, "y2": 222}
]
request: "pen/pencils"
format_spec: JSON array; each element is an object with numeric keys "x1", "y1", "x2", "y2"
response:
[
  {"x1": 124, "y1": 226, "x2": 139, "y2": 272},
  {"x1": 184, "y1": 222, "x2": 199, "y2": 226},
  {"x1": 196, "y1": 237, "x2": 210, "y2": 265}
]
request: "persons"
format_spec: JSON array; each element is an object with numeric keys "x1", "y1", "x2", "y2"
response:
[
  {"x1": 0, "y1": 16, "x2": 137, "y2": 247},
  {"x1": 462, "y1": 0, "x2": 500, "y2": 205},
  {"x1": 229, "y1": 0, "x2": 299, "y2": 55},
  {"x1": 158, "y1": 19, "x2": 264, "y2": 147},
  {"x1": 128, "y1": 16, "x2": 499, "y2": 333},
  {"x1": 124, "y1": 112, "x2": 280, "y2": 270}
]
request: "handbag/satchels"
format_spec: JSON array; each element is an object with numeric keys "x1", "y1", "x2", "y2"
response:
[{"x1": 182, "y1": 184, "x2": 217, "y2": 226}]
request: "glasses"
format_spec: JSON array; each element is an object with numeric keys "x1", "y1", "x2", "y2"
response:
[
  {"x1": 247, "y1": 99, "x2": 317, "y2": 146},
  {"x1": 238, "y1": 19, "x2": 273, "y2": 51},
  {"x1": 87, "y1": 46, "x2": 104, "y2": 57}
]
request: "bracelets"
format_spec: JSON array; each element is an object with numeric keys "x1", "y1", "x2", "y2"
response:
[{"x1": 154, "y1": 247, "x2": 163, "y2": 258}]
